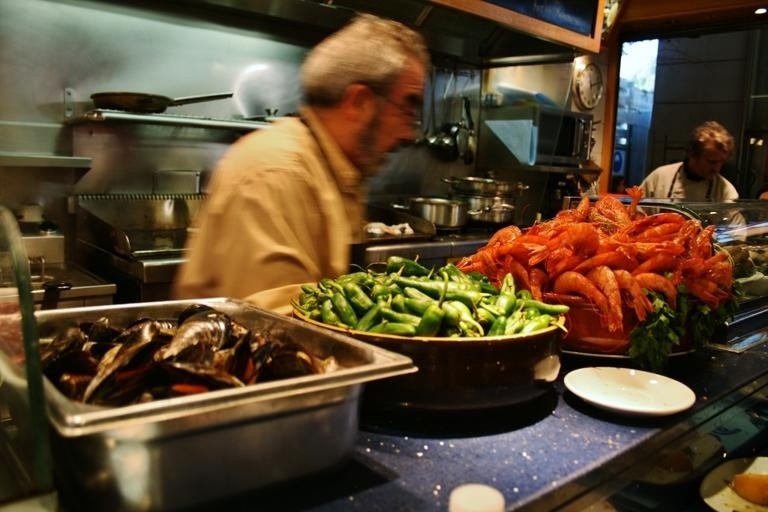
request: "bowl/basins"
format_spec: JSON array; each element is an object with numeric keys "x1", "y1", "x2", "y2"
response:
[
  {"x1": 293, "y1": 290, "x2": 563, "y2": 415},
  {"x1": 542, "y1": 292, "x2": 695, "y2": 358}
]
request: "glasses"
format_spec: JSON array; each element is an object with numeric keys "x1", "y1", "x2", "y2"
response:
[{"x1": 366, "y1": 83, "x2": 423, "y2": 128}]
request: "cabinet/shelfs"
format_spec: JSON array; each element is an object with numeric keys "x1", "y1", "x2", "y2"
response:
[{"x1": 561, "y1": 195, "x2": 768, "y2": 342}]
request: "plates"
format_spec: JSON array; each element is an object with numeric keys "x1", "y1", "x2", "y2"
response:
[
  {"x1": 562, "y1": 366, "x2": 697, "y2": 419},
  {"x1": 559, "y1": 348, "x2": 696, "y2": 359},
  {"x1": 380, "y1": 354, "x2": 562, "y2": 440},
  {"x1": 699, "y1": 456, "x2": 768, "y2": 512}
]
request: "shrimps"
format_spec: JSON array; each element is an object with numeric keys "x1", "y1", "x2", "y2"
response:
[{"x1": 455, "y1": 185, "x2": 737, "y2": 337}]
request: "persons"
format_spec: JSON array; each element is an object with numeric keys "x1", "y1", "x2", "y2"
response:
[
  {"x1": 167, "y1": 13, "x2": 433, "y2": 320},
  {"x1": 638, "y1": 122, "x2": 750, "y2": 243}
]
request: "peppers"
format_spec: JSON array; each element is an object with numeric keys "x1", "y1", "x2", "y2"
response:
[{"x1": 290, "y1": 255, "x2": 569, "y2": 336}]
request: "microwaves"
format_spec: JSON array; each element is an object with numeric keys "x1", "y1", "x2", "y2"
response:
[{"x1": 481, "y1": 104, "x2": 595, "y2": 169}]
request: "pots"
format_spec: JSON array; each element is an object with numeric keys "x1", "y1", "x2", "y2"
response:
[
  {"x1": 391, "y1": 174, "x2": 530, "y2": 232},
  {"x1": 86, "y1": 91, "x2": 234, "y2": 117}
]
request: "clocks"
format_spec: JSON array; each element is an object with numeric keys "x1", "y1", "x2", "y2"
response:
[{"x1": 576, "y1": 60, "x2": 604, "y2": 111}]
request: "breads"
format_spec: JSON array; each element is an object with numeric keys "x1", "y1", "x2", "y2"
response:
[{"x1": 729, "y1": 473, "x2": 768, "y2": 507}]
